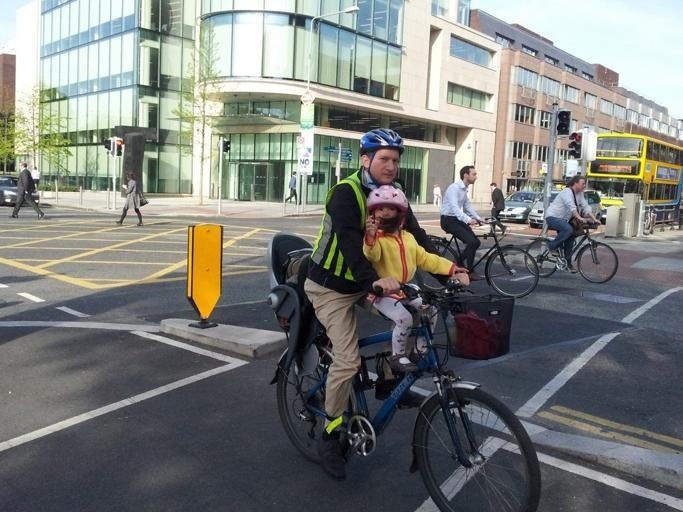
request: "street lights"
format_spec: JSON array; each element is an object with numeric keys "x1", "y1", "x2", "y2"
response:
[{"x1": 302, "y1": 6, "x2": 360, "y2": 215}]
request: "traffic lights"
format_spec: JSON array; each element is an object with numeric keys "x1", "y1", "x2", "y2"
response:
[
  {"x1": 557, "y1": 111, "x2": 570, "y2": 135},
  {"x1": 115, "y1": 138, "x2": 124, "y2": 157},
  {"x1": 568, "y1": 132, "x2": 582, "y2": 159},
  {"x1": 104, "y1": 139, "x2": 112, "y2": 150},
  {"x1": 223, "y1": 140, "x2": 231, "y2": 152}
]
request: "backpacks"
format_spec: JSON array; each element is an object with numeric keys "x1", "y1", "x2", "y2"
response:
[{"x1": 280, "y1": 246, "x2": 314, "y2": 287}]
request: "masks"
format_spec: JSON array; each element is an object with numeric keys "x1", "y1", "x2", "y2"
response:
[{"x1": 374, "y1": 214, "x2": 400, "y2": 234}]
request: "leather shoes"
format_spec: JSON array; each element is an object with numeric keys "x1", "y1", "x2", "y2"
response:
[
  {"x1": 316, "y1": 431, "x2": 347, "y2": 481},
  {"x1": 373, "y1": 377, "x2": 426, "y2": 408}
]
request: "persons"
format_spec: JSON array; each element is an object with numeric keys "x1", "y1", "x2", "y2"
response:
[
  {"x1": 301, "y1": 126, "x2": 469, "y2": 481},
  {"x1": 543, "y1": 175, "x2": 601, "y2": 274},
  {"x1": 31, "y1": 166, "x2": 39, "y2": 190},
  {"x1": 8, "y1": 161, "x2": 44, "y2": 220},
  {"x1": 113, "y1": 172, "x2": 144, "y2": 226},
  {"x1": 432, "y1": 184, "x2": 440, "y2": 207},
  {"x1": 361, "y1": 185, "x2": 470, "y2": 373},
  {"x1": 393, "y1": 178, "x2": 405, "y2": 195},
  {"x1": 439, "y1": 165, "x2": 485, "y2": 282},
  {"x1": 487, "y1": 182, "x2": 507, "y2": 239},
  {"x1": 284, "y1": 170, "x2": 297, "y2": 205}
]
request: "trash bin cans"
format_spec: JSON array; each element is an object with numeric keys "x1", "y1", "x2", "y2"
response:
[{"x1": 604, "y1": 193, "x2": 640, "y2": 238}]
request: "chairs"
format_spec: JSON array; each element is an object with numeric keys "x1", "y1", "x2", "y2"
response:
[{"x1": 265, "y1": 232, "x2": 323, "y2": 334}]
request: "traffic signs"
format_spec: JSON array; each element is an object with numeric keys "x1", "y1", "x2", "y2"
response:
[{"x1": 324, "y1": 145, "x2": 352, "y2": 162}]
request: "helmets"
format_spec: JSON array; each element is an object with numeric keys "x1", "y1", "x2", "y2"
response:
[
  {"x1": 366, "y1": 184, "x2": 409, "y2": 226},
  {"x1": 359, "y1": 127, "x2": 405, "y2": 156}
]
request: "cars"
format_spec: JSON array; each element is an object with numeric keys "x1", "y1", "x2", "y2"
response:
[
  {"x1": 498, "y1": 189, "x2": 602, "y2": 228},
  {"x1": 0, "y1": 174, "x2": 39, "y2": 207}
]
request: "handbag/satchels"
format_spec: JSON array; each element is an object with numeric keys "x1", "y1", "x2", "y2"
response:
[
  {"x1": 139, "y1": 193, "x2": 149, "y2": 206},
  {"x1": 568, "y1": 216, "x2": 585, "y2": 237}
]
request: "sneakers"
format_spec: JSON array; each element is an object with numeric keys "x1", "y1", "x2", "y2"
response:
[
  {"x1": 38, "y1": 212, "x2": 44, "y2": 220},
  {"x1": 468, "y1": 271, "x2": 483, "y2": 282},
  {"x1": 566, "y1": 265, "x2": 578, "y2": 273},
  {"x1": 389, "y1": 353, "x2": 419, "y2": 374},
  {"x1": 501, "y1": 226, "x2": 508, "y2": 235},
  {"x1": 137, "y1": 222, "x2": 143, "y2": 226},
  {"x1": 9, "y1": 214, "x2": 18, "y2": 219},
  {"x1": 116, "y1": 221, "x2": 123, "y2": 225},
  {"x1": 549, "y1": 245, "x2": 559, "y2": 257}
]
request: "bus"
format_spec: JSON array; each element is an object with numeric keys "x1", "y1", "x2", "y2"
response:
[{"x1": 585, "y1": 132, "x2": 683, "y2": 225}]
